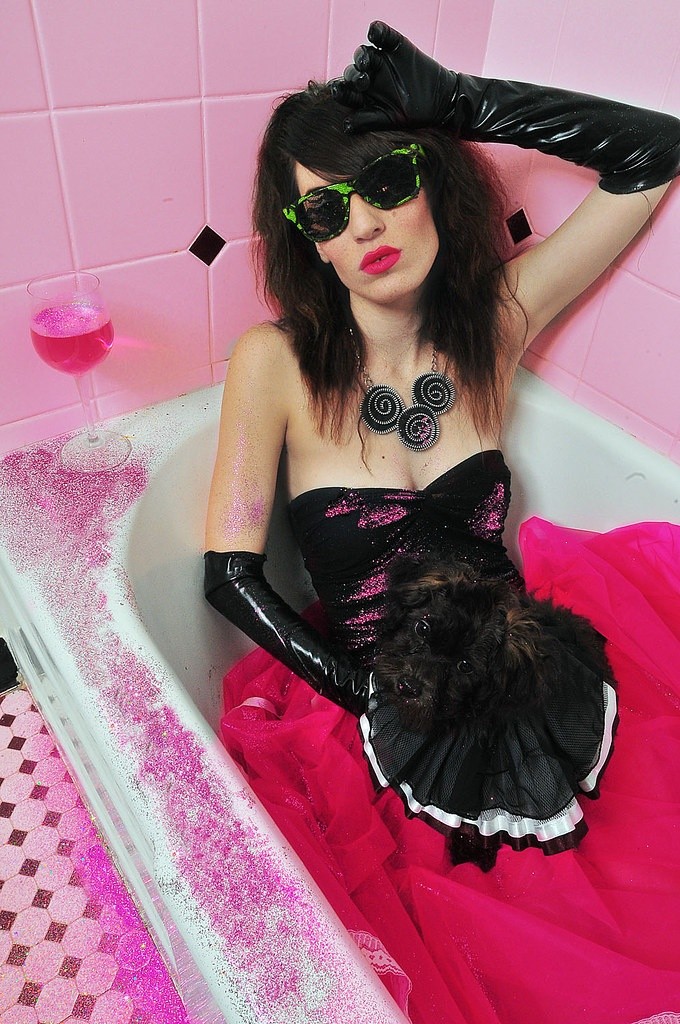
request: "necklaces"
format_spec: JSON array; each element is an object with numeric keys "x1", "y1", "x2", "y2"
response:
[{"x1": 345, "y1": 322, "x2": 457, "y2": 453}]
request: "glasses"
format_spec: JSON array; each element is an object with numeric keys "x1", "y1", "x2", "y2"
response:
[{"x1": 282, "y1": 142, "x2": 425, "y2": 244}]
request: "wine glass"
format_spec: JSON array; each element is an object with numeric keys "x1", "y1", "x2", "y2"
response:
[{"x1": 27, "y1": 270, "x2": 132, "y2": 470}]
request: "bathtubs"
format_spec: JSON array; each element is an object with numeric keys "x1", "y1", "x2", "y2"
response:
[{"x1": 0, "y1": 351, "x2": 680, "y2": 1024}]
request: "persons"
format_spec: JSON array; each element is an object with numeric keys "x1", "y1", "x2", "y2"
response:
[{"x1": 204, "y1": 22, "x2": 680, "y2": 1024}]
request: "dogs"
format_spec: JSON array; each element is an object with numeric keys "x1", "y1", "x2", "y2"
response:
[{"x1": 370, "y1": 554, "x2": 562, "y2": 742}]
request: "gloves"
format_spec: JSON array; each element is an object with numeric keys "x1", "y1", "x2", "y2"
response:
[
  {"x1": 203, "y1": 550, "x2": 374, "y2": 719},
  {"x1": 332, "y1": 20, "x2": 680, "y2": 196}
]
